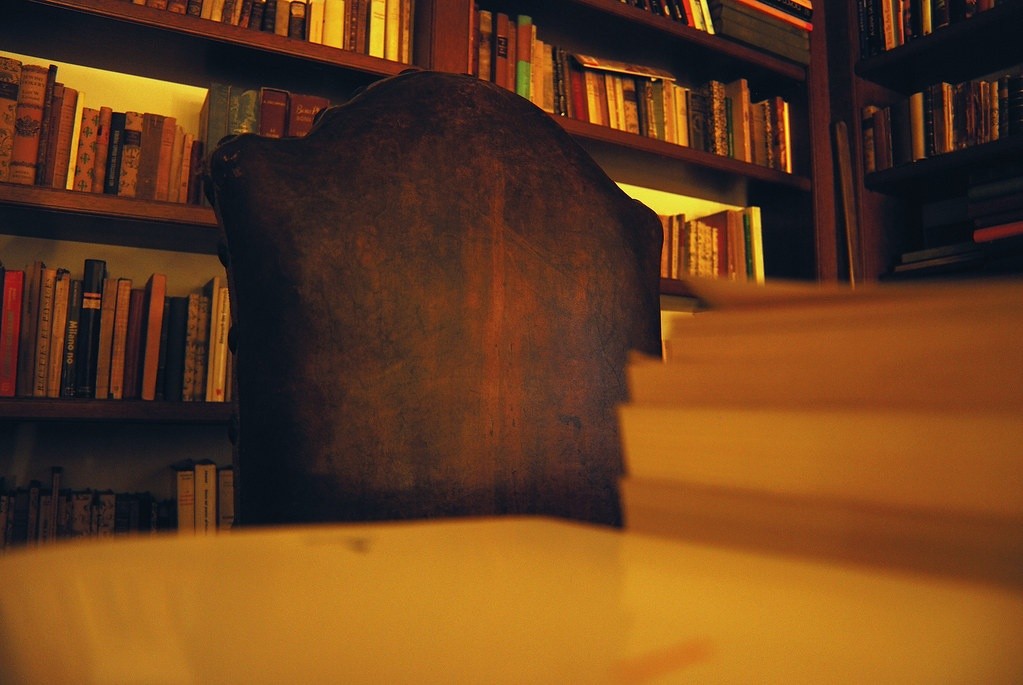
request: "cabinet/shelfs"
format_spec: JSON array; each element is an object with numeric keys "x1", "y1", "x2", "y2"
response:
[{"x1": 0, "y1": 0, "x2": 1023, "y2": 424}]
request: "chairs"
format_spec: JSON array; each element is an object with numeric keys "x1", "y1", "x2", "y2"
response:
[{"x1": 201, "y1": 69, "x2": 670, "y2": 546}]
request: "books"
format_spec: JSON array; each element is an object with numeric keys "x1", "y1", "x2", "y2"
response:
[{"x1": 0, "y1": 1, "x2": 1023, "y2": 684}]
